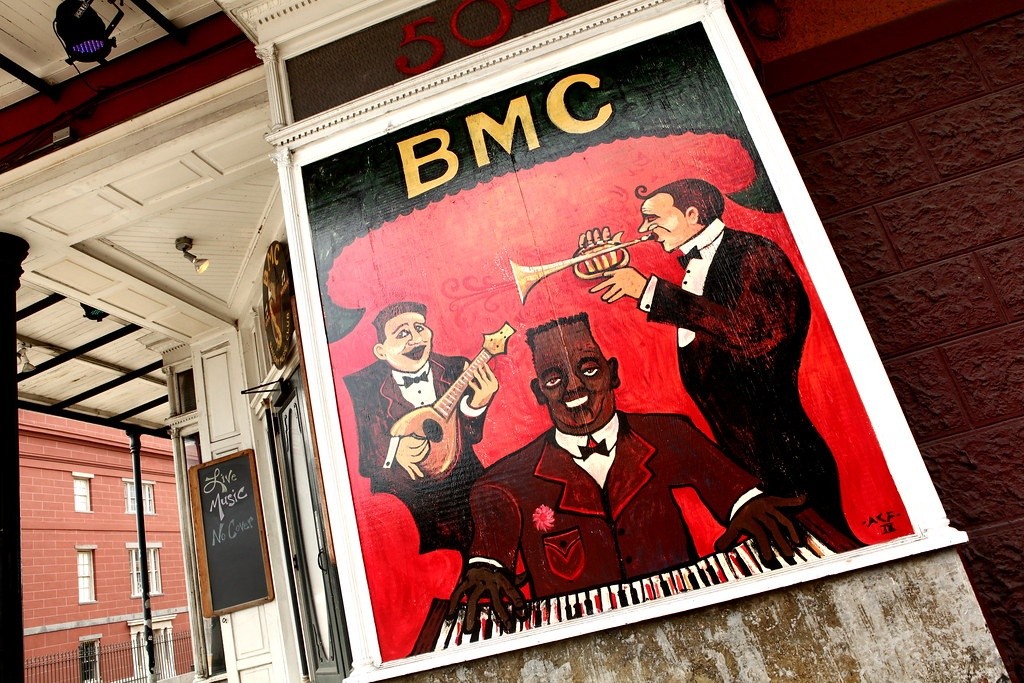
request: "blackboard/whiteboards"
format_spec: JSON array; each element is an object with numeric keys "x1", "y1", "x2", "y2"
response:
[{"x1": 188, "y1": 447, "x2": 275, "y2": 618}]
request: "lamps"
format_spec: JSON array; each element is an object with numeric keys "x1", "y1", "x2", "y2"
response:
[
  {"x1": 176, "y1": 234, "x2": 210, "y2": 274},
  {"x1": 52, "y1": 1, "x2": 126, "y2": 71}
]
